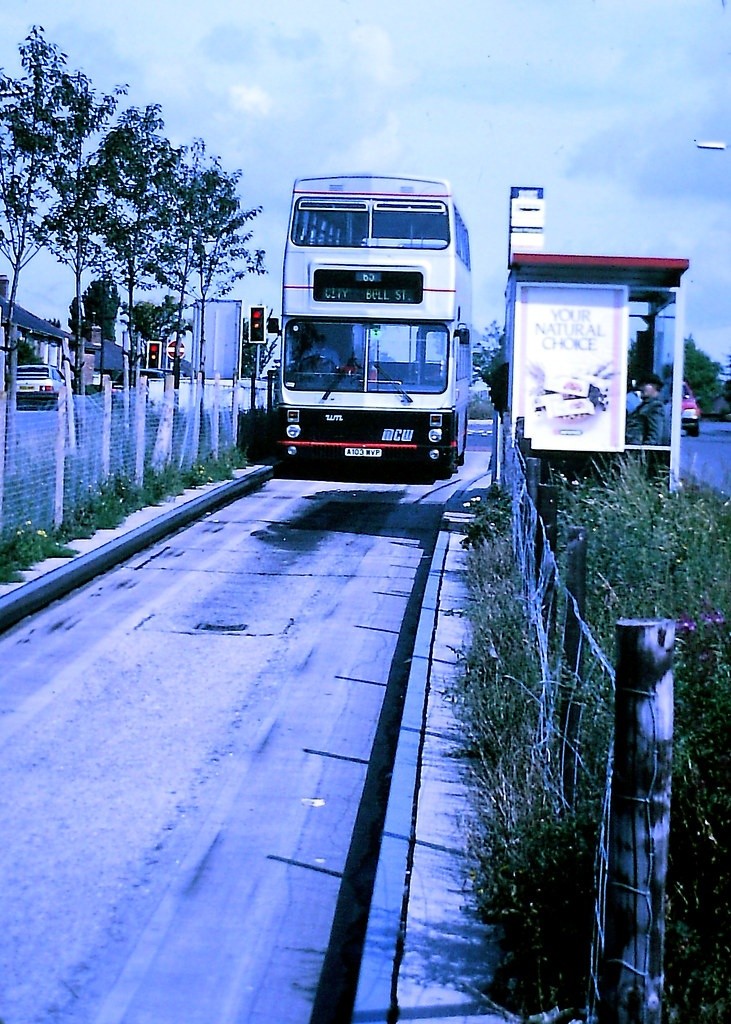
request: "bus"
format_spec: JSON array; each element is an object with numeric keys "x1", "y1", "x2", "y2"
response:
[{"x1": 266, "y1": 172, "x2": 473, "y2": 481}]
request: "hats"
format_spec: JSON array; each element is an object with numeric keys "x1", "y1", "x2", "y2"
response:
[{"x1": 636, "y1": 374, "x2": 664, "y2": 388}]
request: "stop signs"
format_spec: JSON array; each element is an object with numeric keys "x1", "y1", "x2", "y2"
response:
[{"x1": 166, "y1": 341, "x2": 186, "y2": 359}]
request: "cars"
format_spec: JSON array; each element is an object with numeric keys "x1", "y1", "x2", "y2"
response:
[
  {"x1": 681, "y1": 382, "x2": 703, "y2": 437},
  {"x1": 15, "y1": 365, "x2": 66, "y2": 393}
]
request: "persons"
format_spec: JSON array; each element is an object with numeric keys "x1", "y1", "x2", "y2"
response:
[
  {"x1": 626, "y1": 373, "x2": 668, "y2": 444},
  {"x1": 299, "y1": 334, "x2": 341, "y2": 382}
]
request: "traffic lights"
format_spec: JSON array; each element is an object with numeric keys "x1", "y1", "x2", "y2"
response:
[
  {"x1": 248, "y1": 305, "x2": 266, "y2": 380},
  {"x1": 145, "y1": 341, "x2": 163, "y2": 369}
]
